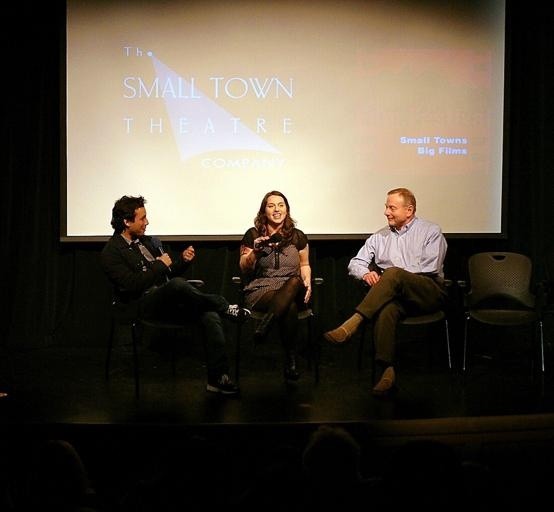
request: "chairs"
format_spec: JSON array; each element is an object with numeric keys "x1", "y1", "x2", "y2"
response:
[
  {"x1": 92, "y1": 259, "x2": 209, "y2": 396},
  {"x1": 373, "y1": 264, "x2": 451, "y2": 368},
  {"x1": 463, "y1": 251, "x2": 543, "y2": 372},
  {"x1": 232, "y1": 258, "x2": 324, "y2": 387}
]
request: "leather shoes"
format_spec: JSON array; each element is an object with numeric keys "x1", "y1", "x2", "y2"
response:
[
  {"x1": 322, "y1": 326, "x2": 349, "y2": 345},
  {"x1": 372, "y1": 376, "x2": 395, "y2": 396}
]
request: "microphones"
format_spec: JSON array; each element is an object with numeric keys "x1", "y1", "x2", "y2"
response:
[
  {"x1": 255, "y1": 233, "x2": 282, "y2": 248},
  {"x1": 151, "y1": 238, "x2": 172, "y2": 272}
]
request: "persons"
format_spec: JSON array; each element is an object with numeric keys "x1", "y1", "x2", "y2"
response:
[
  {"x1": 322, "y1": 187, "x2": 449, "y2": 396},
  {"x1": 239, "y1": 191, "x2": 312, "y2": 380},
  {"x1": 100, "y1": 194, "x2": 251, "y2": 395}
]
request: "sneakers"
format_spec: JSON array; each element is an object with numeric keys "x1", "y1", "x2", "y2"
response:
[
  {"x1": 219, "y1": 304, "x2": 252, "y2": 325},
  {"x1": 206, "y1": 371, "x2": 240, "y2": 395}
]
